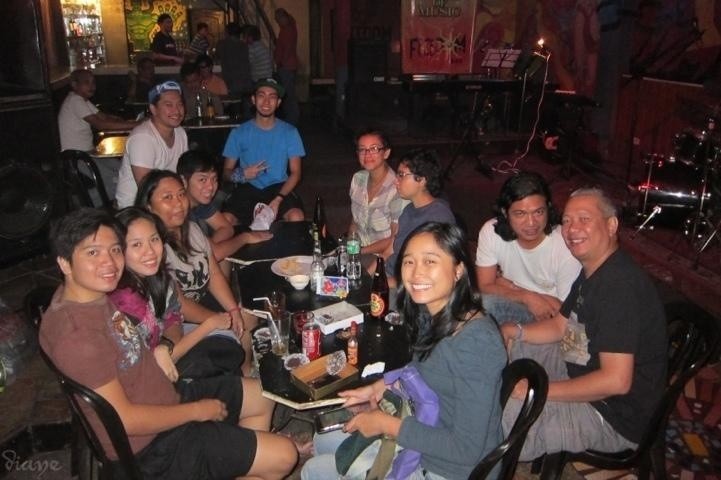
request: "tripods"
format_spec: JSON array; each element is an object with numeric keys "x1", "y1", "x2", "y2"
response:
[
  {"x1": 682, "y1": 167, "x2": 721, "y2": 248},
  {"x1": 549, "y1": 131, "x2": 584, "y2": 186}
]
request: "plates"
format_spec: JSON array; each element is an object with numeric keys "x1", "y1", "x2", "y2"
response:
[
  {"x1": 214, "y1": 115, "x2": 229, "y2": 119},
  {"x1": 253, "y1": 327, "x2": 270, "y2": 341},
  {"x1": 383, "y1": 312, "x2": 404, "y2": 325},
  {"x1": 281, "y1": 352, "x2": 310, "y2": 371},
  {"x1": 269, "y1": 255, "x2": 328, "y2": 276}
]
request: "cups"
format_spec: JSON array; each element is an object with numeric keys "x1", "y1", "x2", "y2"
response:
[
  {"x1": 292, "y1": 309, "x2": 314, "y2": 335},
  {"x1": 265, "y1": 309, "x2": 291, "y2": 355},
  {"x1": 262, "y1": 291, "x2": 286, "y2": 319}
]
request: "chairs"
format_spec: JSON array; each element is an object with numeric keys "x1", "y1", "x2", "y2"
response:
[
  {"x1": 454, "y1": 357, "x2": 549, "y2": 479},
  {"x1": 53, "y1": 150, "x2": 115, "y2": 213},
  {"x1": 528, "y1": 305, "x2": 719, "y2": 480},
  {"x1": 26, "y1": 285, "x2": 146, "y2": 480}
]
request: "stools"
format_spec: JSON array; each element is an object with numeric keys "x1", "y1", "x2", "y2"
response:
[{"x1": 538, "y1": 90, "x2": 603, "y2": 182}]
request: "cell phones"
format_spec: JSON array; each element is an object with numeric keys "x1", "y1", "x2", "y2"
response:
[{"x1": 315, "y1": 401, "x2": 370, "y2": 434}]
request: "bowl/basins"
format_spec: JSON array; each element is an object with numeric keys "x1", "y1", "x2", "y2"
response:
[{"x1": 289, "y1": 275, "x2": 309, "y2": 290}]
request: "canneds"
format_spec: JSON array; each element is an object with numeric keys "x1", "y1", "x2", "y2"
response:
[{"x1": 302, "y1": 323, "x2": 321, "y2": 361}]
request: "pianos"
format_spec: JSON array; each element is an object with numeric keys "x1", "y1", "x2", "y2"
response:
[{"x1": 441, "y1": 80, "x2": 532, "y2": 94}]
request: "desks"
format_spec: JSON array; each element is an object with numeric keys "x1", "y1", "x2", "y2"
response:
[{"x1": 403, "y1": 74, "x2": 523, "y2": 178}]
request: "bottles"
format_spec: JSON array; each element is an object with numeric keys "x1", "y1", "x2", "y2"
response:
[
  {"x1": 310, "y1": 195, "x2": 327, "y2": 293},
  {"x1": 193, "y1": 85, "x2": 215, "y2": 121},
  {"x1": 335, "y1": 227, "x2": 362, "y2": 281},
  {"x1": 345, "y1": 321, "x2": 359, "y2": 365},
  {"x1": 368, "y1": 256, "x2": 389, "y2": 318},
  {"x1": 67, "y1": 16, "x2": 82, "y2": 36}
]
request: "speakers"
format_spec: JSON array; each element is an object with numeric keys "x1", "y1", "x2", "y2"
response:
[{"x1": 0, "y1": 93, "x2": 67, "y2": 269}]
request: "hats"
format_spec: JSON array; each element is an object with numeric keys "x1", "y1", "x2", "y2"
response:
[
  {"x1": 253, "y1": 76, "x2": 280, "y2": 98},
  {"x1": 148, "y1": 80, "x2": 182, "y2": 103}
]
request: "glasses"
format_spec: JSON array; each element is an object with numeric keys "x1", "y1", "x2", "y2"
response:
[
  {"x1": 395, "y1": 170, "x2": 413, "y2": 177},
  {"x1": 356, "y1": 146, "x2": 385, "y2": 154}
]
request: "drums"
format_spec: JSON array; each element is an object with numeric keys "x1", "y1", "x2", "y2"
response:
[
  {"x1": 639, "y1": 154, "x2": 713, "y2": 235},
  {"x1": 676, "y1": 129, "x2": 720, "y2": 166}
]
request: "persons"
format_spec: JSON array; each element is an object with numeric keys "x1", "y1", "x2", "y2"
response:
[
  {"x1": 345, "y1": 128, "x2": 458, "y2": 311},
  {"x1": 135, "y1": 9, "x2": 297, "y2": 120},
  {"x1": 37, "y1": 77, "x2": 336, "y2": 480},
  {"x1": 57, "y1": 69, "x2": 141, "y2": 195},
  {"x1": 303, "y1": 222, "x2": 509, "y2": 480},
  {"x1": 474, "y1": 172, "x2": 669, "y2": 463}
]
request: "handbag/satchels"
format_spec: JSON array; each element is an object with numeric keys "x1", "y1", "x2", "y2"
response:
[{"x1": 334, "y1": 366, "x2": 440, "y2": 480}]
